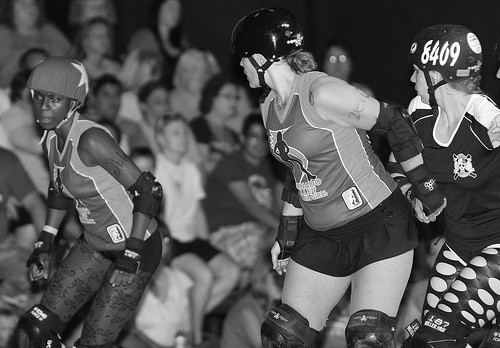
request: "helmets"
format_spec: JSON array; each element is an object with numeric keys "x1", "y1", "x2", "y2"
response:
[
  {"x1": 231, "y1": 7, "x2": 304, "y2": 61},
  {"x1": 408, "y1": 24, "x2": 482, "y2": 82},
  {"x1": 27, "y1": 57, "x2": 89, "y2": 109}
]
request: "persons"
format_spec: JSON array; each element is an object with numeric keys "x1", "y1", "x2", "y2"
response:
[
  {"x1": 230, "y1": 8, "x2": 447, "y2": 348},
  {"x1": 0, "y1": 0, "x2": 375, "y2": 348},
  {"x1": 16, "y1": 57, "x2": 163, "y2": 348},
  {"x1": 387, "y1": 25, "x2": 500, "y2": 348}
]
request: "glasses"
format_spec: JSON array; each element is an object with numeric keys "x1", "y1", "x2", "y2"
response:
[
  {"x1": 220, "y1": 93, "x2": 240, "y2": 102},
  {"x1": 329, "y1": 55, "x2": 348, "y2": 63}
]
